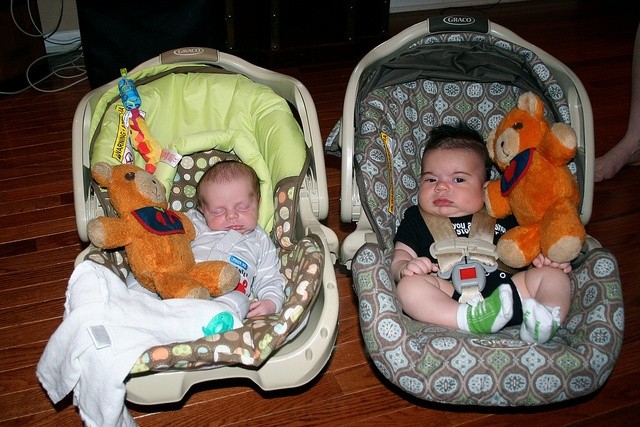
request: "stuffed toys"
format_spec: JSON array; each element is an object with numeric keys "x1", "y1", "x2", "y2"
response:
[
  {"x1": 128, "y1": 110, "x2": 162, "y2": 173},
  {"x1": 88, "y1": 161, "x2": 240, "y2": 300},
  {"x1": 483, "y1": 93, "x2": 586, "y2": 268}
]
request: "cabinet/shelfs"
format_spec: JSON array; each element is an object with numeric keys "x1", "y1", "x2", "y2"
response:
[{"x1": 74, "y1": 0, "x2": 389, "y2": 89}]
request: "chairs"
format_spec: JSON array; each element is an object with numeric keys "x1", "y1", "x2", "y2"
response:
[
  {"x1": 352, "y1": 82, "x2": 624, "y2": 409},
  {"x1": 71, "y1": 45, "x2": 340, "y2": 409}
]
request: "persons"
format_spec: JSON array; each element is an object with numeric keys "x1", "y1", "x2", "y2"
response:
[
  {"x1": 124, "y1": 161, "x2": 285, "y2": 324},
  {"x1": 594, "y1": 97, "x2": 640, "y2": 182},
  {"x1": 393, "y1": 123, "x2": 571, "y2": 343}
]
